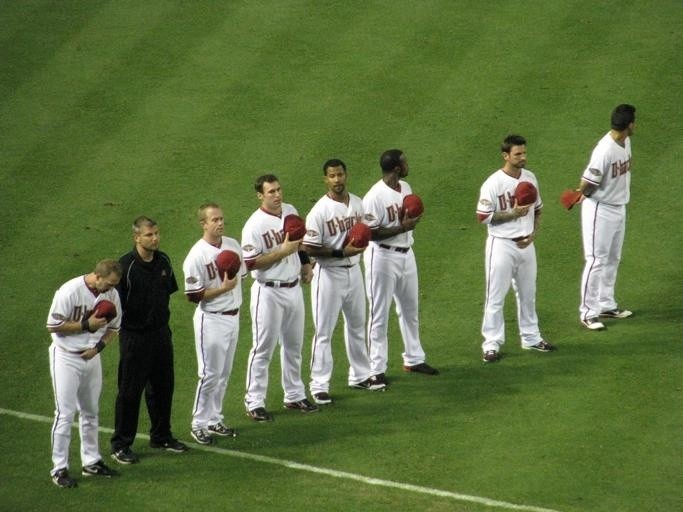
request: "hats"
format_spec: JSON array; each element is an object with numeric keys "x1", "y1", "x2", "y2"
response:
[
  {"x1": 399, "y1": 194, "x2": 424, "y2": 224},
  {"x1": 341, "y1": 222, "x2": 371, "y2": 251},
  {"x1": 512, "y1": 182, "x2": 537, "y2": 207},
  {"x1": 283, "y1": 215, "x2": 305, "y2": 242},
  {"x1": 84, "y1": 300, "x2": 115, "y2": 332},
  {"x1": 560, "y1": 188, "x2": 581, "y2": 210},
  {"x1": 215, "y1": 250, "x2": 241, "y2": 281}
]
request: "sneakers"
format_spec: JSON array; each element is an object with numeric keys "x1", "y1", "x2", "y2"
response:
[
  {"x1": 599, "y1": 307, "x2": 632, "y2": 318},
  {"x1": 371, "y1": 373, "x2": 388, "y2": 385},
  {"x1": 312, "y1": 391, "x2": 331, "y2": 404},
  {"x1": 283, "y1": 399, "x2": 317, "y2": 411},
  {"x1": 51, "y1": 468, "x2": 76, "y2": 487},
  {"x1": 207, "y1": 422, "x2": 233, "y2": 436},
  {"x1": 521, "y1": 339, "x2": 552, "y2": 352},
  {"x1": 349, "y1": 378, "x2": 385, "y2": 391},
  {"x1": 246, "y1": 407, "x2": 272, "y2": 422},
  {"x1": 403, "y1": 362, "x2": 439, "y2": 376},
  {"x1": 581, "y1": 317, "x2": 604, "y2": 330},
  {"x1": 110, "y1": 446, "x2": 138, "y2": 465},
  {"x1": 483, "y1": 350, "x2": 497, "y2": 362},
  {"x1": 152, "y1": 439, "x2": 186, "y2": 453},
  {"x1": 190, "y1": 429, "x2": 213, "y2": 444},
  {"x1": 81, "y1": 459, "x2": 117, "y2": 476}
]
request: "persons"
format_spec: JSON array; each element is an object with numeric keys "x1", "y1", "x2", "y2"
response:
[
  {"x1": 43, "y1": 257, "x2": 124, "y2": 490},
  {"x1": 110, "y1": 214, "x2": 188, "y2": 466},
  {"x1": 181, "y1": 200, "x2": 250, "y2": 447},
  {"x1": 573, "y1": 102, "x2": 636, "y2": 332},
  {"x1": 302, "y1": 158, "x2": 387, "y2": 404},
  {"x1": 474, "y1": 133, "x2": 557, "y2": 364},
  {"x1": 361, "y1": 148, "x2": 439, "y2": 388},
  {"x1": 240, "y1": 173, "x2": 321, "y2": 424}
]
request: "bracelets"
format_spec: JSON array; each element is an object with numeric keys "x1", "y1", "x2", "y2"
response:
[
  {"x1": 94, "y1": 338, "x2": 106, "y2": 354},
  {"x1": 330, "y1": 247, "x2": 344, "y2": 260},
  {"x1": 79, "y1": 318, "x2": 90, "y2": 332},
  {"x1": 504, "y1": 206, "x2": 520, "y2": 221},
  {"x1": 399, "y1": 222, "x2": 407, "y2": 234},
  {"x1": 296, "y1": 247, "x2": 312, "y2": 267}
]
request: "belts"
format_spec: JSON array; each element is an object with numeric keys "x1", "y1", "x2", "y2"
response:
[
  {"x1": 379, "y1": 244, "x2": 410, "y2": 253},
  {"x1": 265, "y1": 278, "x2": 298, "y2": 288},
  {"x1": 210, "y1": 308, "x2": 238, "y2": 316},
  {"x1": 511, "y1": 235, "x2": 530, "y2": 242}
]
